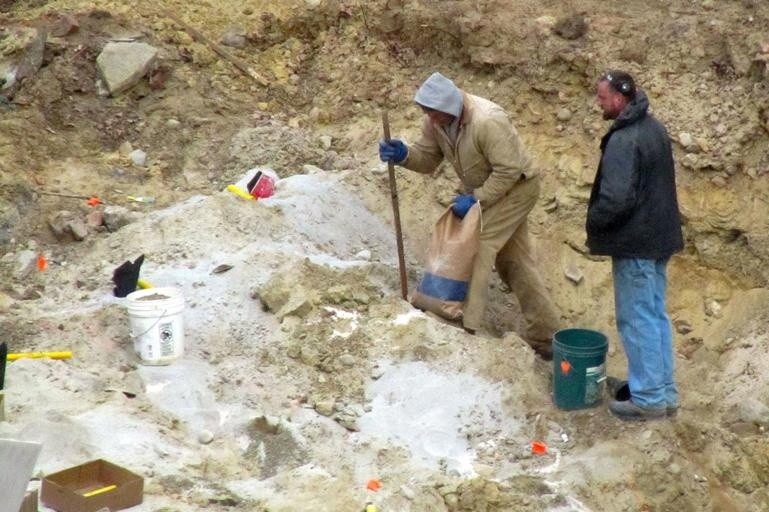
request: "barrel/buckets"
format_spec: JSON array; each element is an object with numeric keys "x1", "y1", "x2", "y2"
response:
[
  {"x1": 552, "y1": 328, "x2": 609, "y2": 410},
  {"x1": 126, "y1": 286, "x2": 185, "y2": 365}
]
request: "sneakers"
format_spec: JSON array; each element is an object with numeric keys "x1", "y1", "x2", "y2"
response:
[
  {"x1": 608, "y1": 397, "x2": 679, "y2": 422},
  {"x1": 533, "y1": 344, "x2": 554, "y2": 361}
]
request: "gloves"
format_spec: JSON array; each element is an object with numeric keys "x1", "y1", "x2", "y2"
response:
[
  {"x1": 378, "y1": 138, "x2": 410, "y2": 165},
  {"x1": 448, "y1": 193, "x2": 474, "y2": 221}
]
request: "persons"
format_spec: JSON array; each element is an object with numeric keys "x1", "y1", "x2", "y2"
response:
[
  {"x1": 583, "y1": 69, "x2": 687, "y2": 422},
  {"x1": 379, "y1": 71, "x2": 564, "y2": 359}
]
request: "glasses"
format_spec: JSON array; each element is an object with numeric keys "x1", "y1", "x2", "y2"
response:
[{"x1": 604, "y1": 70, "x2": 622, "y2": 90}]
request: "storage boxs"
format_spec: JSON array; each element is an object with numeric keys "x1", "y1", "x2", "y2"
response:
[{"x1": 39, "y1": 457, "x2": 143, "y2": 511}]
